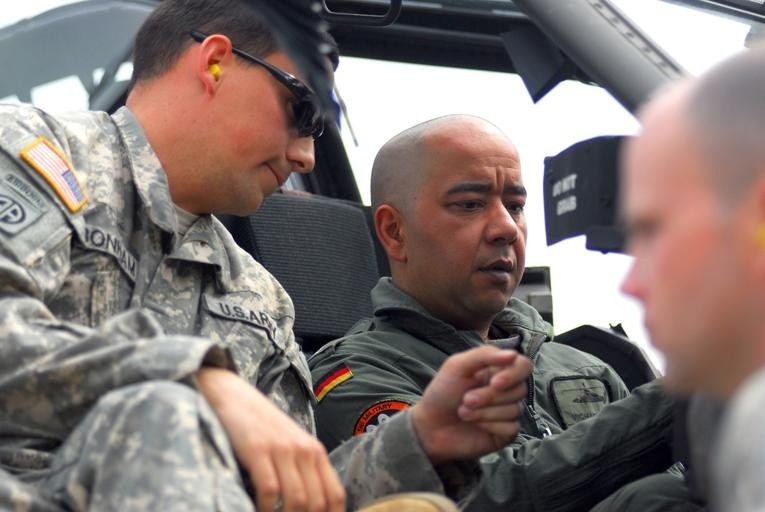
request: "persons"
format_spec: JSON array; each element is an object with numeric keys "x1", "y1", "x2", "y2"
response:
[
  {"x1": 619, "y1": 46, "x2": 764, "y2": 511},
  {"x1": 306, "y1": 112, "x2": 702, "y2": 510},
  {"x1": 1, "y1": 1, "x2": 534, "y2": 512}
]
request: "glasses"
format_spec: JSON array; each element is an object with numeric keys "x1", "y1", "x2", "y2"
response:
[{"x1": 193, "y1": 30, "x2": 324, "y2": 138}]
